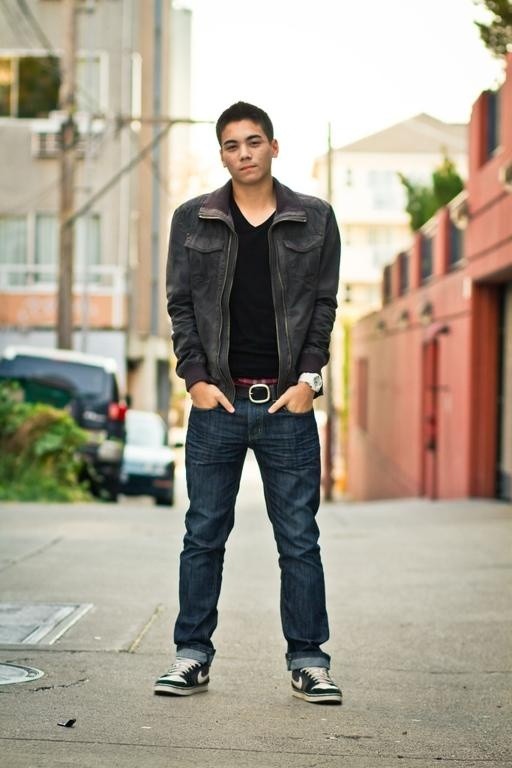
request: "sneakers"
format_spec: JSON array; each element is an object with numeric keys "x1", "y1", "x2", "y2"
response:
[
  {"x1": 291, "y1": 666, "x2": 342, "y2": 702},
  {"x1": 154, "y1": 659, "x2": 209, "y2": 696}
]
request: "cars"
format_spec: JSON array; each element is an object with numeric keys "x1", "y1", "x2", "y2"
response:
[{"x1": 117, "y1": 410, "x2": 183, "y2": 505}]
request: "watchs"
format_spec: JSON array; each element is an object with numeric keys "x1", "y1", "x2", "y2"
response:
[{"x1": 298, "y1": 372, "x2": 323, "y2": 393}]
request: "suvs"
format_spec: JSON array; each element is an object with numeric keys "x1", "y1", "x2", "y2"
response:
[{"x1": 1, "y1": 349, "x2": 126, "y2": 503}]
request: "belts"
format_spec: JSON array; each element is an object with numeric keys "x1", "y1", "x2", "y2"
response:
[{"x1": 234, "y1": 383, "x2": 279, "y2": 403}]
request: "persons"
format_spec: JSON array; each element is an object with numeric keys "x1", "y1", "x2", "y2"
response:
[{"x1": 153, "y1": 100, "x2": 342, "y2": 703}]
